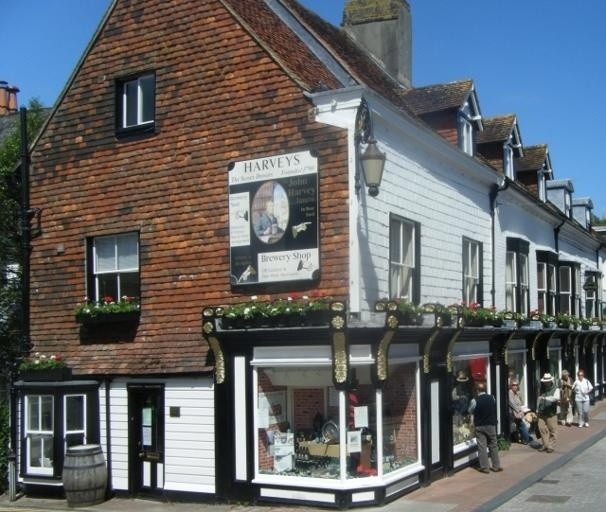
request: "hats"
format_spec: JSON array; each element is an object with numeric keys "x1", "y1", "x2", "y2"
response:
[{"x1": 541, "y1": 372, "x2": 554, "y2": 382}]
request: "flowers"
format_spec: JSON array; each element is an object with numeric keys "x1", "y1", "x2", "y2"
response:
[
  {"x1": 556, "y1": 313, "x2": 592, "y2": 324},
  {"x1": 75, "y1": 295, "x2": 141, "y2": 314},
  {"x1": 533, "y1": 311, "x2": 555, "y2": 324},
  {"x1": 19, "y1": 352, "x2": 68, "y2": 370},
  {"x1": 223, "y1": 291, "x2": 330, "y2": 320},
  {"x1": 390, "y1": 297, "x2": 425, "y2": 316},
  {"x1": 464, "y1": 303, "x2": 502, "y2": 321}
]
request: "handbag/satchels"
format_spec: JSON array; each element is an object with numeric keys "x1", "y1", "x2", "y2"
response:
[{"x1": 539, "y1": 400, "x2": 557, "y2": 416}]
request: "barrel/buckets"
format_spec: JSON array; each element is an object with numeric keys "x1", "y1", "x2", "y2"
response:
[{"x1": 61, "y1": 444, "x2": 109, "y2": 508}]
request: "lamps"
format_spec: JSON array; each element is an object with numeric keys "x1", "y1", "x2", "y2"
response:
[{"x1": 359, "y1": 97, "x2": 386, "y2": 196}]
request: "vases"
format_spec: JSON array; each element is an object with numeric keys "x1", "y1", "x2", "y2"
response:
[
  {"x1": 20, "y1": 368, "x2": 72, "y2": 381},
  {"x1": 75, "y1": 313, "x2": 140, "y2": 323},
  {"x1": 573, "y1": 322, "x2": 577, "y2": 329},
  {"x1": 582, "y1": 323, "x2": 589, "y2": 330},
  {"x1": 388, "y1": 311, "x2": 416, "y2": 326},
  {"x1": 558, "y1": 320, "x2": 569, "y2": 327},
  {"x1": 222, "y1": 312, "x2": 323, "y2": 328},
  {"x1": 542, "y1": 321, "x2": 549, "y2": 327},
  {"x1": 470, "y1": 316, "x2": 501, "y2": 328}
]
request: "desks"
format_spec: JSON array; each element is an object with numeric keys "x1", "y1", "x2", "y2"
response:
[{"x1": 300, "y1": 441, "x2": 350, "y2": 468}]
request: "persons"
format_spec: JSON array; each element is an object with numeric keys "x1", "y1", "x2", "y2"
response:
[
  {"x1": 508, "y1": 368, "x2": 517, "y2": 385},
  {"x1": 572, "y1": 370, "x2": 594, "y2": 428},
  {"x1": 535, "y1": 372, "x2": 561, "y2": 453},
  {"x1": 254, "y1": 197, "x2": 285, "y2": 237},
  {"x1": 451, "y1": 371, "x2": 475, "y2": 433},
  {"x1": 557, "y1": 369, "x2": 574, "y2": 426},
  {"x1": 468, "y1": 384, "x2": 504, "y2": 473},
  {"x1": 508, "y1": 378, "x2": 535, "y2": 445}
]
